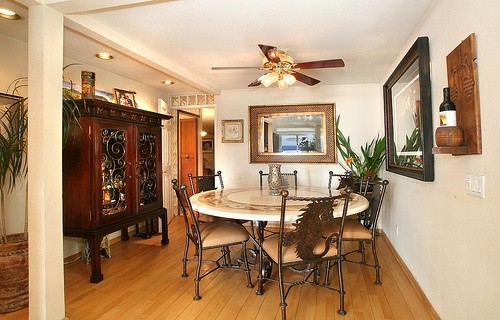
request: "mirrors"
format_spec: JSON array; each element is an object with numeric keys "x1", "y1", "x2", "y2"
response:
[
  {"x1": 249, "y1": 103, "x2": 336, "y2": 163},
  {"x1": 383, "y1": 37, "x2": 434, "y2": 182}
]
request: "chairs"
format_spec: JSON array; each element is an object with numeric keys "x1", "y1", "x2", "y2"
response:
[{"x1": 171, "y1": 170, "x2": 389, "y2": 320}]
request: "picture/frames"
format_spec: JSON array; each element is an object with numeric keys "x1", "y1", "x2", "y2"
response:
[
  {"x1": 221, "y1": 119, "x2": 244, "y2": 143},
  {"x1": 202, "y1": 139, "x2": 213, "y2": 153},
  {"x1": 114, "y1": 88, "x2": 137, "y2": 108},
  {"x1": 0, "y1": 93, "x2": 24, "y2": 163}
]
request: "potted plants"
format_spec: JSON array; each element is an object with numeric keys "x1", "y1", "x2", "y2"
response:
[
  {"x1": 336, "y1": 114, "x2": 386, "y2": 192},
  {"x1": 0, "y1": 62, "x2": 83, "y2": 314}
]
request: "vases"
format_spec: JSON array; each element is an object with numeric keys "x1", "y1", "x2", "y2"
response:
[{"x1": 268, "y1": 164, "x2": 282, "y2": 195}]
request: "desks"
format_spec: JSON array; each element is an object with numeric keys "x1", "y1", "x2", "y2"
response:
[{"x1": 189, "y1": 187, "x2": 370, "y2": 283}]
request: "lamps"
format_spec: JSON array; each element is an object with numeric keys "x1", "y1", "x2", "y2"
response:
[{"x1": 258, "y1": 65, "x2": 296, "y2": 90}]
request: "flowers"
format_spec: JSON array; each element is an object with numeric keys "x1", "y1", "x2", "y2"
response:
[{"x1": 394, "y1": 100, "x2": 423, "y2": 169}]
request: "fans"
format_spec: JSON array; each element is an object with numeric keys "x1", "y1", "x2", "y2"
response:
[{"x1": 211, "y1": 44, "x2": 345, "y2": 87}]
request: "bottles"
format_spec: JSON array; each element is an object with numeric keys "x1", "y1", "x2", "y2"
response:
[{"x1": 439, "y1": 87, "x2": 457, "y2": 127}]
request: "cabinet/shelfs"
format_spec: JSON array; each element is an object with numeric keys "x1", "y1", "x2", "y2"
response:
[{"x1": 63, "y1": 99, "x2": 169, "y2": 284}]
